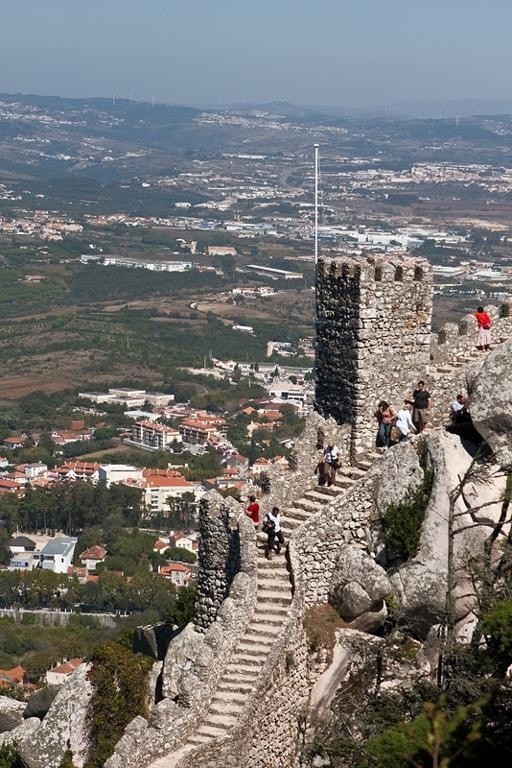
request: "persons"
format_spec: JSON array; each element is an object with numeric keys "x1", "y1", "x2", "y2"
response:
[
  {"x1": 376, "y1": 401, "x2": 394, "y2": 450},
  {"x1": 394, "y1": 403, "x2": 417, "y2": 440},
  {"x1": 320, "y1": 441, "x2": 341, "y2": 488},
  {"x1": 246, "y1": 494, "x2": 260, "y2": 531},
  {"x1": 449, "y1": 394, "x2": 467, "y2": 420},
  {"x1": 412, "y1": 381, "x2": 431, "y2": 435},
  {"x1": 262, "y1": 506, "x2": 284, "y2": 560},
  {"x1": 471, "y1": 306, "x2": 493, "y2": 351}
]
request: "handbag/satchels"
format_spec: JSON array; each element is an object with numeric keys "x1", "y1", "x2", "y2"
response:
[
  {"x1": 393, "y1": 418, "x2": 397, "y2": 427},
  {"x1": 262, "y1": 520, "x2": 275, "y2": 536},
  {"x1": 332, "y1": 459, "x2": 341, "y2": 469}
]
